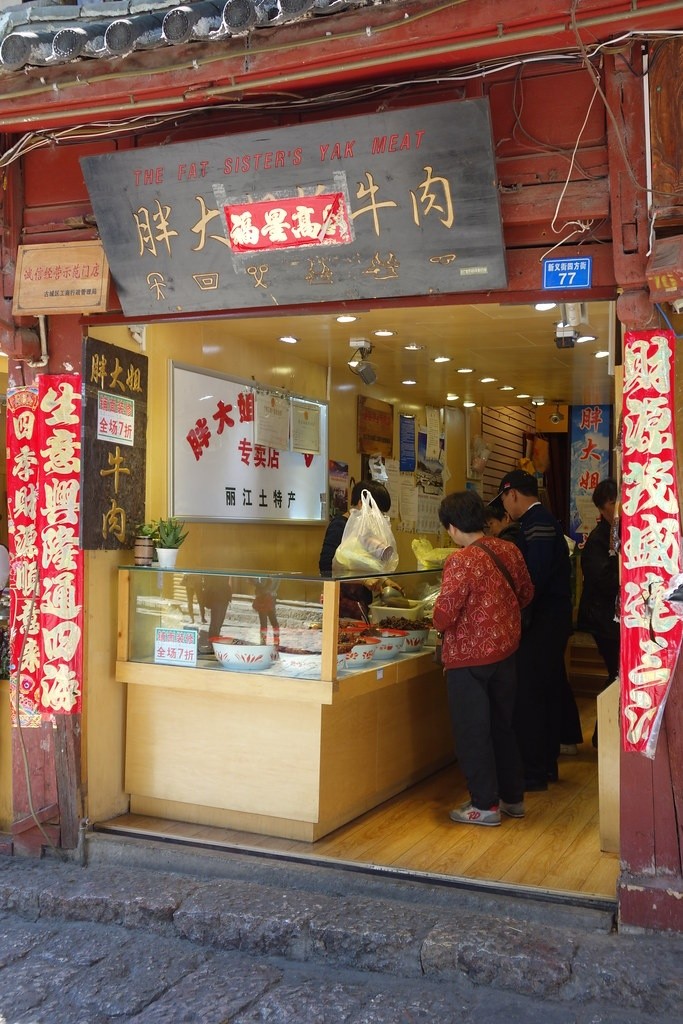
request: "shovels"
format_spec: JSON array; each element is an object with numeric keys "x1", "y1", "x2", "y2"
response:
[{"x1": 380, "y1": 586, "x2": 411, "y2": 608}]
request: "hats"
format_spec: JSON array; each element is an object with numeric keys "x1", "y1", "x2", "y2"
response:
[{"x1": 487, "y1": 468, "x2": 539, "y2": 507}]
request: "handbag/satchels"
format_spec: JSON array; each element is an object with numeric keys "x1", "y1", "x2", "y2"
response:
[{"x1": 334, "y1": 488, "x2": 399, "y2": 574}]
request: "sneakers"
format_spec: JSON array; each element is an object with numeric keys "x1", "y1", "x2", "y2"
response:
[
  {"x1": 561, "y1": 743, "x2": 578, "y2": 755},
  {"x1": 448, "y1": 804, "x2": 503, "y2": 827},
  {"x1": 499, "y1": 799, "x2": 525, "y2": 818}
]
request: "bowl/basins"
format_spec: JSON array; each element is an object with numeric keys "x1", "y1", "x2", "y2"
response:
[{"x1": 208, "y1": 621, "x2": 432, "y2": 669}]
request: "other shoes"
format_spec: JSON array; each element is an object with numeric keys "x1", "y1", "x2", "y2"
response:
[
  {"x1": 530, "y1": 779, "x2": 549, "y2": 793},
  {"x1": 547, "y1": 771, "x2": 559, "y2": 783}
]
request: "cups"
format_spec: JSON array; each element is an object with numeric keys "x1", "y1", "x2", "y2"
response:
[
  {"x1": 135, "y1": 536, "x2": 153, "y2": 566},
  {"x1": 360, "y1": 533, "x2": 393, "y2": 560}
]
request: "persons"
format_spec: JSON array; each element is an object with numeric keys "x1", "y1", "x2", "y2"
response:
[
  {"x1": 578, "y1": 477, "x2": 620, "y2": 751},
  {"x1": 432, "y1": 490, "x2": 536, "y2": 827},
  {"x1": 318, "y1": 479, "x2": 408, "y2": 615},
  {"x1": 178, "y1": 573, "x2": 283, "y2": 648},
  {"x1": 486, "y1": 469, "x2": 585, "y2": 793}
]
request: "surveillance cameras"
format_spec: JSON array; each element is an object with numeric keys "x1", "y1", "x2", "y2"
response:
[
  {"x1": 552, "y1": 416, "x2": 560, "y2": 424},
  {"x1": 356, "y1": 361, "x2": 376, "y2": 385},
  {"x1": 561, "y1": 303, "x2": 589, "y2": 328}
]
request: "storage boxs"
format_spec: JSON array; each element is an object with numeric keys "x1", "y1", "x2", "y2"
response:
[{"x1": 369, "y1": 598, "x2": 425, "y2": 625}]
